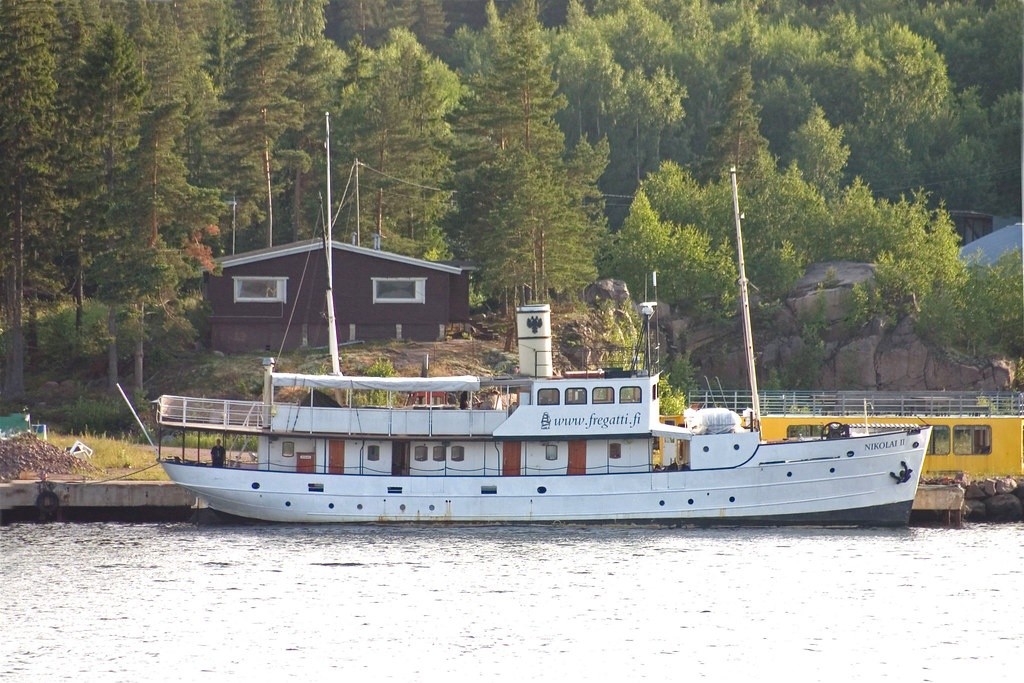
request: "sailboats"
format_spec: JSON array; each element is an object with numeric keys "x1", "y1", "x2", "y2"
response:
[{"x1": 115, "y1": 109, "x2": 935, "y2": 528}]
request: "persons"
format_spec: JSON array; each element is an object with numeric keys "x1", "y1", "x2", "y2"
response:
[
  {"x1": 459, "y1": 391, "x2": 468, "y2": 410},
  {"x1": 211, "y1": 439, "x2": 226, "y2": 468}
]
